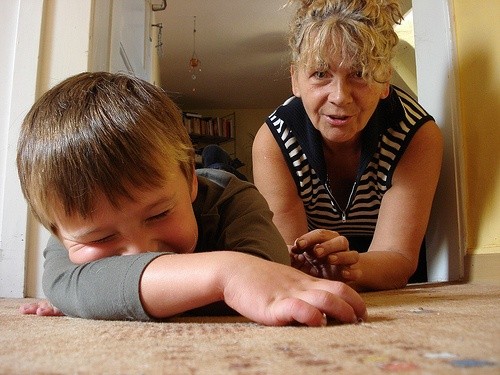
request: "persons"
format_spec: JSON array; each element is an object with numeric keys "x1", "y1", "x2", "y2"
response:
[
  {"x1": 252, "y1": 0, "x2": 442, "y2": 291},
  {"x1": 16, "y1": 72, "x2": 369, "y2": 328}
]
